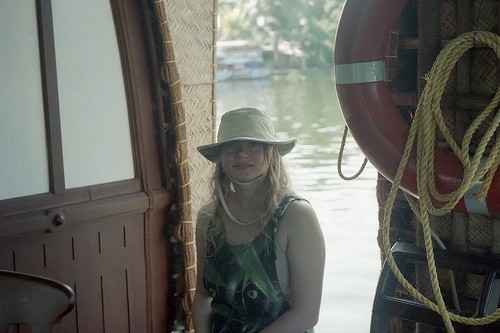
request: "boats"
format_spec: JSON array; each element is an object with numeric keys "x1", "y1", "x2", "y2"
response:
[{"x1": 214, "y1": 56, "x2": 271, "y2": 82}]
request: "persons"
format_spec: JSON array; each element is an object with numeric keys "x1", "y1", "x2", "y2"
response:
[{"x1": 188, "y1": 106, "x2": 326, "y2": 333}]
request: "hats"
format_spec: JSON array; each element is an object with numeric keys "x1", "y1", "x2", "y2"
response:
[{"x1": 196, "y1": 107, "x2": 297, "y2": 163}]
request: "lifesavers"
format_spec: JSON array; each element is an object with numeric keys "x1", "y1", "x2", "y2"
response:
[{"x1": 332, "y1": 0, "x2": 500, "y2": 220}]
